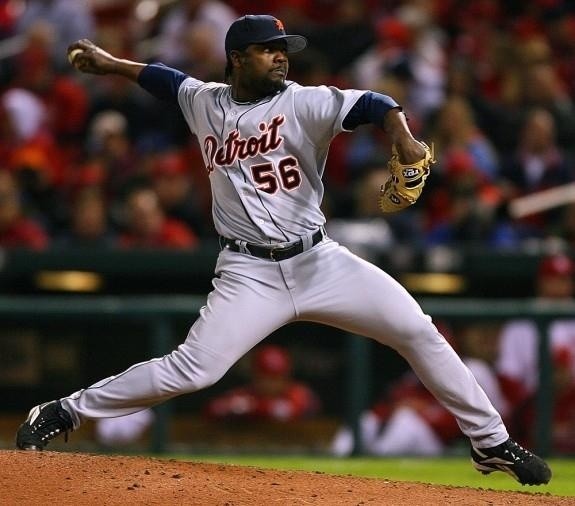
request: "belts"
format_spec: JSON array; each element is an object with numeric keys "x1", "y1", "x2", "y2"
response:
[{"x1": 221, "y1": 227, "x2": 327, "y2": 262}]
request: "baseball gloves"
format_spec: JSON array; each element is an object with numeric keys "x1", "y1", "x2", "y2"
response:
[{"x1": 377, "y1": 141, "x2": 436, "y2": 213}]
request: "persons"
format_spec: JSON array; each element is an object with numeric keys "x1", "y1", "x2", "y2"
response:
[
  {"x1": 2, "y1": 2, "x2": 575, "y2": 456},
  {"x1": 15, "y1": 11, "x2": 557, "y2": 485}
]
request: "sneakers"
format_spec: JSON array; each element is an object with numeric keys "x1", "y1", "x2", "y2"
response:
[
  {"x1": 14, "y1": 397, "x2": 75, "y2": 452},
  {"x1": 467, "y1": 431, "x2": 554, "y2": 488}
]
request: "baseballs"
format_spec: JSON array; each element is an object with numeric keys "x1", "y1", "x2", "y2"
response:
[{"x1": 68, "y1": 48, "x2": 84, "y2": 64}]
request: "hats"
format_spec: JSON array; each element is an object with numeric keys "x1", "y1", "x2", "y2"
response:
[{"x1": 224, "y1": 12, "x2": 308, "y2": 57}]
